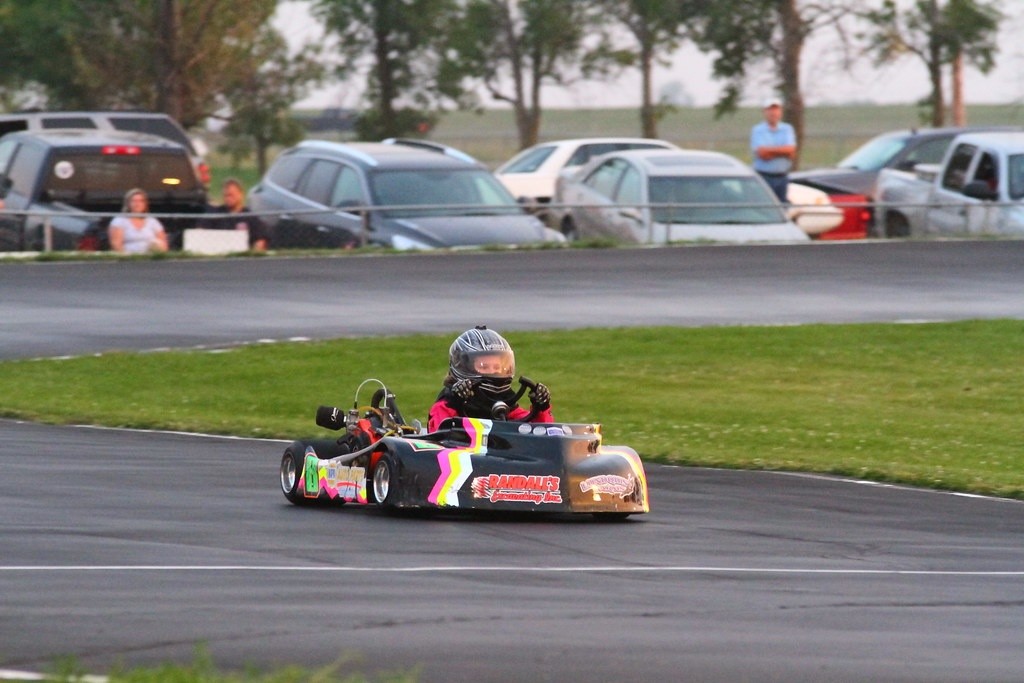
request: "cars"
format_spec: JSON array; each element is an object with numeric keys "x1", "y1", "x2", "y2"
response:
[
  {"x1": 786, "y1": 125, "x2": 1024, "y2": 244},
  {"x1": 496, "y1": 135, "x2": 684, "y2": 225},
  {"x1": 552, "y1": 145, "x2": 812, "y2": 244}
]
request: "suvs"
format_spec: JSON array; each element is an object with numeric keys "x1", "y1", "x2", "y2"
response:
[
  {"x1": 0, "y1": 130, "x2": 210, "y2": 259},
  {"x1": 245, "y1": 132, "x2": 574, "y2": 251}
]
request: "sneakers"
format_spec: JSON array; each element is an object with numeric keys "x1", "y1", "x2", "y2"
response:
[{"x1": 448, "y1": 326, "x2": 516, "y2": 398}]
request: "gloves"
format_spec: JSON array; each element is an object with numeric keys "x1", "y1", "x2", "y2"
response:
[
  {"x1": 527, "y1": 382, "x2": 550, "y2": 412},
  {"x1": 451, "y1": 378, "x2": 474, "y2": 403}
]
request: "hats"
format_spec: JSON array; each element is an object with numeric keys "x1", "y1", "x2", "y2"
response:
[{"x1": 764, "y1": 99, "x2": 783, "y2": 109}]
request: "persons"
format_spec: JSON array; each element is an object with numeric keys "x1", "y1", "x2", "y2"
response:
[
  {"x1": 427, "y1": 326, "x2": 554, "y2": 434},
  {"x1": 750, "y1": 98, "x2": 797, "y2": 202},
  {"x1": 197, "y1": 179, "x2": 270, "y2": 250},
  {"x1": 108, "y1": 187, "x2": 169, "y2": 254}
]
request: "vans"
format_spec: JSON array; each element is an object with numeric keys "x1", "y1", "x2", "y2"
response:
[{"x1": 0, "y1": 110, "x2": 210, "y2": 188}]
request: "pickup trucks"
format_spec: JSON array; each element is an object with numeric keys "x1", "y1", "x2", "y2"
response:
[{"x1": 874, "y1": 134, "x2": 1024, "y2": 240}]
